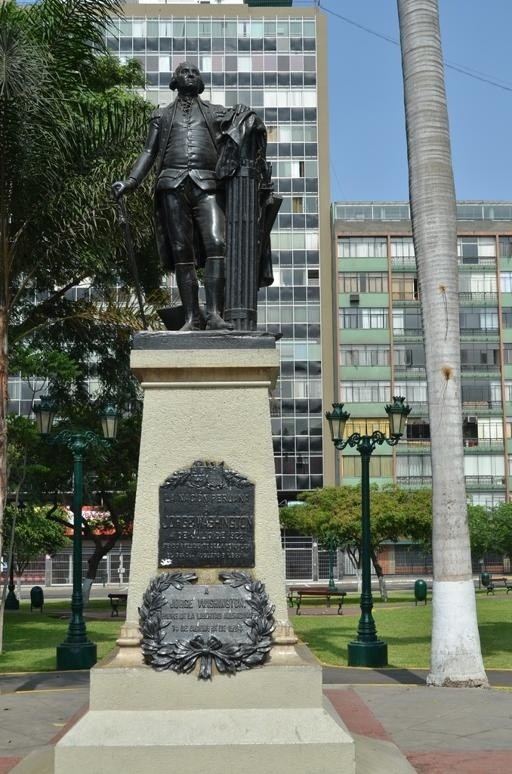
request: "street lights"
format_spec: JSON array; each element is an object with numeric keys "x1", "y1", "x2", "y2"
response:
[
  {"x1": 26, "y1": 384, "x2": 126, "y2": 672},
  {"x1": 3, "y1": 499, "x2": 26, "y2": 609},
  {"x1": 325, "y1": 396, "x2": 413, "y2": 667},
  {"x1": 322, "y1": 539, "x2": 339, "y2": 593}
]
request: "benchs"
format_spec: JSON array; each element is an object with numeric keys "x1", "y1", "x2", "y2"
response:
[
  {"x1": 108, "y1": 594, "x2": 127, "y2": 617},
  {"x1": 293, "y1": 592, "x2": 346, "y2": 615},
  {"x1": 286, "y1": 587, "x2": 330, "y2": 608},
  {"x1": 486, "y1": 577, "x2": 512, "y2": 595}
]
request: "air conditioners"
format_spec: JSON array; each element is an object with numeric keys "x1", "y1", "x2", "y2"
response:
[{"x1": 467, "y1": 415, "x2": 478, "y2": 423}]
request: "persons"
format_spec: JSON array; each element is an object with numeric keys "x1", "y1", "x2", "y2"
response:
[{"x1": 111, "y1": 62, "x2": 250, "y2": 331}]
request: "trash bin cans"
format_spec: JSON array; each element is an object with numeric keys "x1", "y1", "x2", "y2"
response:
[
  {"x1": 414, "y1": 579, "x2": 427, "y2": 607},
  {"x1": 481, "y1": 572, "x2": 489, "y2": 587},
  {"x1": 29, "y1": 586, "x2": 44, "y2": 614}
]
